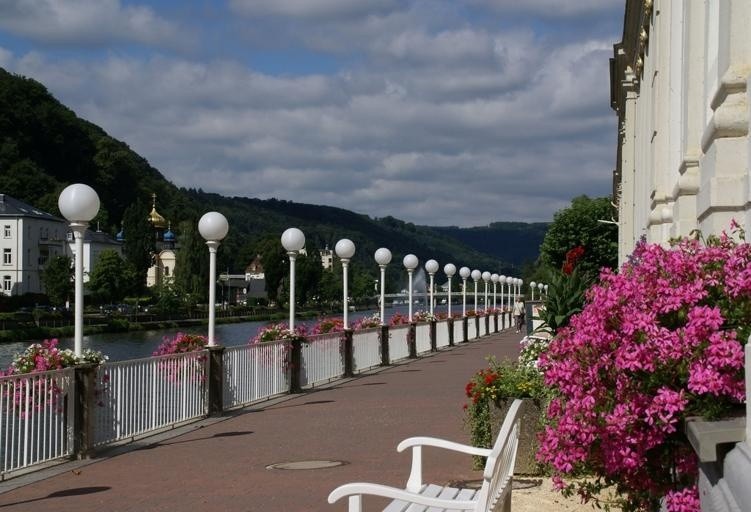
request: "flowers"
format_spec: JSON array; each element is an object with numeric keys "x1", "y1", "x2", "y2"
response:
[
  {"x1": 1, "y1": 336, "x2": 112, "y2": 422},
  {"x1": 463, "y1": 230, "x2": 750, "y2": 511},
  {"x1": 151, "y1": 331, "x2": 220, "y2": 387},
  {"x1": 248, "y1": 322, "x2": 312, "y2": 371},
  {"x1": 312, "y1": 310, "x2": 433, "y2": 342}
]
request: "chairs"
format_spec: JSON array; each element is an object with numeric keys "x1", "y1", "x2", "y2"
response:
[{"x1": 326, "y1": 398, "x2": 524, "y2": 512}]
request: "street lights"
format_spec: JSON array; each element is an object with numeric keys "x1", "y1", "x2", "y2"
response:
[
  {"x1": 375, "y1": 247, "x2": 393, "y2": 325},
  {"x1": 58, "y1": 184, "x2": 101, "y2": 358},
  {"x1": 459, "y1": 266, "x2": 471, "y2": 318},
  {"x1": 336, "y1": 239, "x2": 356, "y2": 330},
  {"x1": 198, "y1": 211, "x2": 229, "y2": 347},
  {"x1": 482, "y1": 271, "x2": 525, "y2": 313},
  {"x1": 424, "y1": 258, "x2": 439, "y2": 317},
  {"x1": 472, "y1": 269, "x2": 482, "y2": 315},
  {"x1": 531, "y1": 281, "x2": 549, "y2": 301},
  {"x1": 281, "y1": 227, "x2": 307, "y2": 337},
  {"x1": 444, "y1": 263, "x2": 457, "y2": 318},
  {"x1": 404, "y1": 253, "x2": 420, "y2": 323}
]
